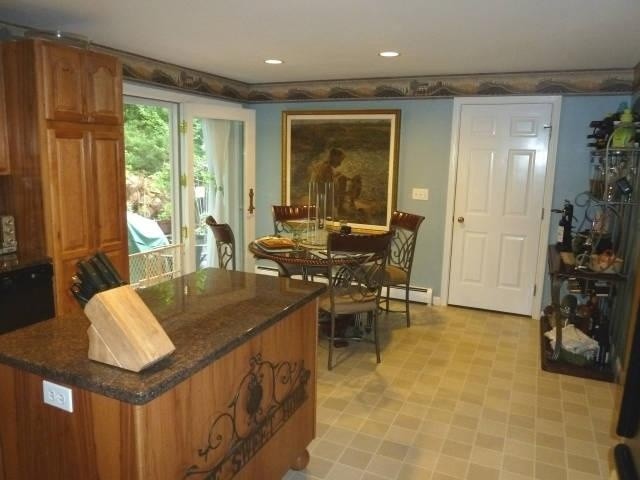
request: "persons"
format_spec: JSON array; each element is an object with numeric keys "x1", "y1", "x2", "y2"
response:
[{"x1": 311, "y1": 147, "x2": 362, "y2": 217}]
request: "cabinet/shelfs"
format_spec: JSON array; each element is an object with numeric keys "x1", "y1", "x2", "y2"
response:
[
  {"x1": 0, "y1": 40, "x2": 130, "y2": 317},
  {"x1": 540, "y1": 122, "x2": 640, "y2": 383}
]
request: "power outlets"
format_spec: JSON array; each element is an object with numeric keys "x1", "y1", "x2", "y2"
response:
[{"x1": 43, "y1": 381, "x2": 73, "y2": 416}]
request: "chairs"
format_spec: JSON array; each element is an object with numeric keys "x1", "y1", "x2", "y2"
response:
[
  {"x1": 206, "y1": 216, "x2": 236, "y2": 271},
  {"x1": 271, "y1": 206, "x2": 425, "y2": 371}
]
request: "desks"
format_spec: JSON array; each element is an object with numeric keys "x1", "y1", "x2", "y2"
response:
[{"x1": 249, "y1": 235, "x2": 392, "y2": 279}]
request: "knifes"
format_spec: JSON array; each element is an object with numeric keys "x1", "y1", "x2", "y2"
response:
[{"x1": 69, "y1": 252, "x2": 127, "y2": 306}]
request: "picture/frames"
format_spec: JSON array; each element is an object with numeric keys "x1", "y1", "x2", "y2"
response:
[{"x1": 282, "y1": 110, "x2": 401, "y2": 235}]
